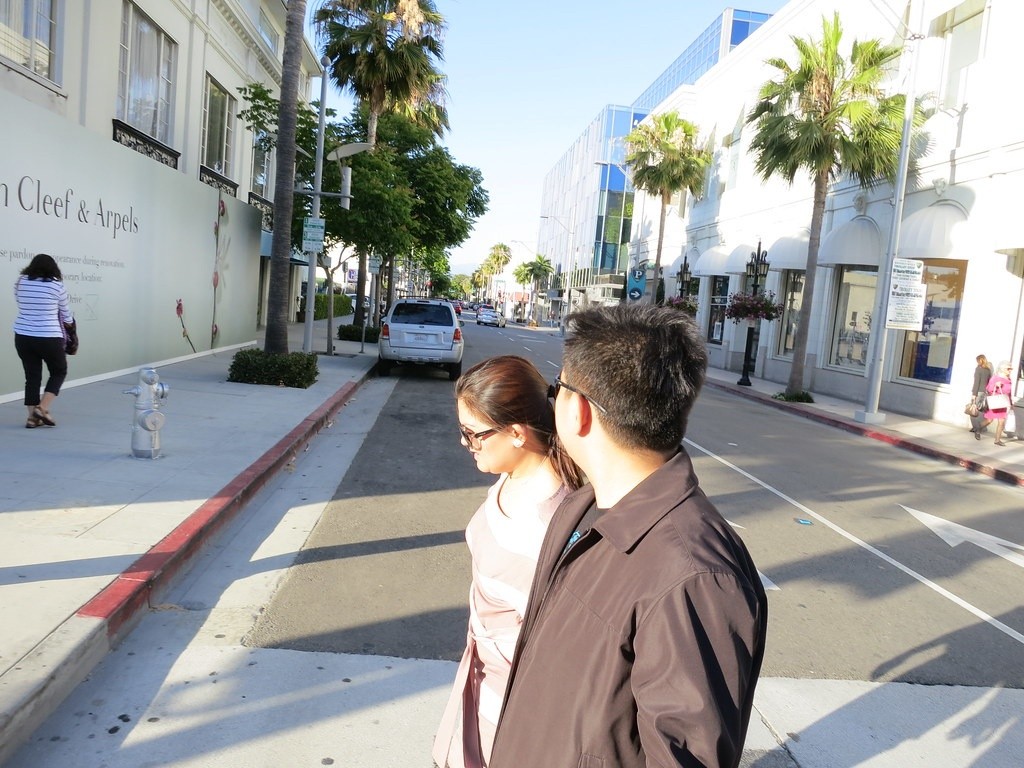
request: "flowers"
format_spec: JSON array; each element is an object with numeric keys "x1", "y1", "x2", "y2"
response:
[
  {"x1": 667, "y1": 296, "x2": 698, "y2": 314},
  {"x1": 723, "y1": 290, "x2": 786, "y2": 323}
]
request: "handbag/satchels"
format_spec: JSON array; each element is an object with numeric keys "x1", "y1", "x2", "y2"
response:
[
  {"x1": 1002, "y1": 408, "x2": 1015, "y2": 433},
  {"x1": 977, "y1": 386, "x2": 990, "y2": 411},
  {"x1": 987, "y1": 384, "x2": 1011, "y2": 409},
  {"x1": 64, "y1": 317, "x2": 78, "y2": 355},
  {"x1": 965, "y1": 399, "x2": 978, "y2": 417}
]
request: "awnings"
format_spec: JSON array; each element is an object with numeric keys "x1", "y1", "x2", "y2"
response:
[
  {"x1": 724, "y1": 238, "x2": 763, "y2": 276},
  {"x1": 816, "y1": 219, "x2": 881, "y2": 268},
  {"x1": 765, "y1": 228, "x2": 812, "y2": 272},
  {"x1": 894, "y1": 205, "x2": 972, "y2": 259},
  {"x1": 669, "y1": 247, "x2": 700, "y2": 279},
  {"x1": 692, "y1": 240, "x2": 732, "y2": 278}
]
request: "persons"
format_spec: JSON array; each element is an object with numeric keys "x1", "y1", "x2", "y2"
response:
[
  {"x1": 431, "y1": 352, "x2": 587, "y2": 768},
  {"x1": 14, "y1": 253, "x2": 73, "y2": 428},
  {"x1": 970, "y1": 361, "x2": 1017, "y2": 446},
  {"x1": 966, "y1": 354, "x2": 990, "y2": 440},
  {"x1": 488, "y1": 304, "x2": 769, "y2": 768}
]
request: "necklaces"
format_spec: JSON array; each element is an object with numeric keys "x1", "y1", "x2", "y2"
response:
[{"x1": 508, "y1": 455, "x2": 547, "y2": 480}]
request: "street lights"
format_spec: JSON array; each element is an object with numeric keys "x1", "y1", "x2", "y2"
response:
[
  {"x1": 736, "y1": 240, "x2": 771, "y2": 386},
  {"x1": 594, "y1": 159, "x2": 647, "y2": 276},
  {"x1": 675, "y1": 255, "x2": 692, "y2": 299},
  {"x1": 539, "y1": 216, "x2": 574, "y2": 328},
  {"x1": 784, "y1": 271, "x2": 803, "y2": 349}
]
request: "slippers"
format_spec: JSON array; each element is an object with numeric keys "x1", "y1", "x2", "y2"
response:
[
  {"x1": 994, "y1": 440, "x2": 1005, "y2": 447},
  {"x1": 976, "y1": 431, "x2": 980, "y2": 441}
]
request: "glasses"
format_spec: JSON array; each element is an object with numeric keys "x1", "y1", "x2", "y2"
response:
[
  {"x1": 1005, "y1": 368, "x2": 1013, "y2": 371},
  {"x1": 554, "y1": 374, "x2": 608, "y2": 415},
  {"x1": 459, "y1": 418, "x2": 531, "y2": 446}
]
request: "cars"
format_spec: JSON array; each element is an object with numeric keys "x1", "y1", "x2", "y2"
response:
[
  {"x1": 377, "y1": 295, "x2": 465, "y2": 381},
  {"x1": 476, "y1": 310, "x2": 507, "y2": 328},
  {"x1": 434, "y1": 298, "x2": 496, "y2": 315},
  {"x1": 344, "y1": 293, "x2": 385, "y2": 314}
]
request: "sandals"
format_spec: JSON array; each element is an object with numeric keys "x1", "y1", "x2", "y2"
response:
[
  {"x1": 26, "y1": 418, "x2": 44, "y2": 428},
  {"x1": 33, "y1": 405, "x2": 56, "y2": 426}
]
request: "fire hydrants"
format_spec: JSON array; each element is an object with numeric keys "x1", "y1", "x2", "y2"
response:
[{"x1": 121, "y1": 367, "x2": 169, "y2": 461}]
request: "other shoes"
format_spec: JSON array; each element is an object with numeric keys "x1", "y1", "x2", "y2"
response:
[{"x1": 971, "y1": 428, "x2": 975, "y2": 432}]
